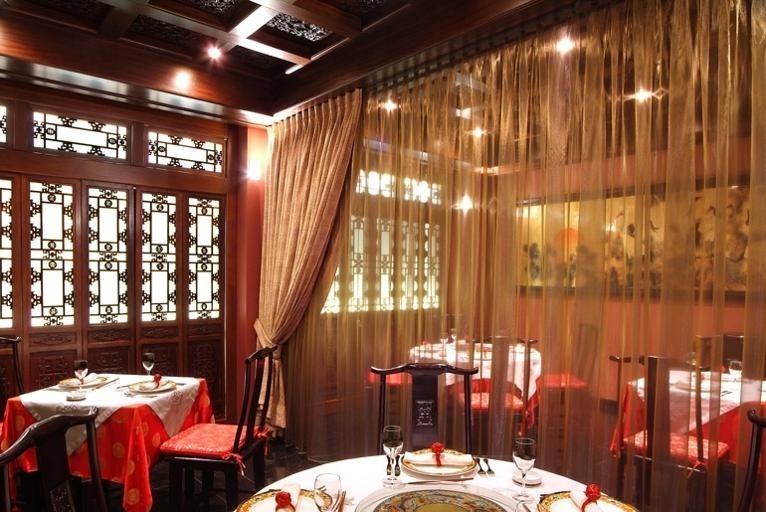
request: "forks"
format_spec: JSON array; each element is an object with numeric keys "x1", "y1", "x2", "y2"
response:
[{"x1": 476, "y1": 458, "x2": 496, "y2": 480}]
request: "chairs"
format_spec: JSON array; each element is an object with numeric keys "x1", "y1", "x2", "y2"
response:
[
  {"x1": 0, "y1": 335, "x2": 108, "y2": 512},
  {"x1": 609, "y1": 335, "x2": 766, "y2": 511},
  {"x1": 159, "y1": 344, "x2": 277, "y2": 512},
  {"x1": 370, "y1": 309, "x2": 598, "y2": 455}
]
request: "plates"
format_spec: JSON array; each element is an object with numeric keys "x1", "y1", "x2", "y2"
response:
[
  {"x1": 538, "y1": 490, "x2": 637, "y2": 512},
  {"x1": 128, "y1": 383, "x2": 176, "y2": 393},
  {"x1": 58, "y1": 375, "x2": 104, "y2": 389},
  {"x1": 232, "y1": 488, "x2": 335, "y2": 512},
  {"x1": 404, "y1": 448, "x2": 475, "y2": 477}
]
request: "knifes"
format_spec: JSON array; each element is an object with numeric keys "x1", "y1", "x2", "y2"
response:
[{"x1": 96, "y1": 377, "x2": 121, "y2": 389}]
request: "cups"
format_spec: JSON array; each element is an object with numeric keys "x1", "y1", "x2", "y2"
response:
[
  {"x1": 450, "y1": 328, "x2": 458, "y2": 341},
  {"x1": 314, "y1": 474, "x2": 344, "y2": 512}
]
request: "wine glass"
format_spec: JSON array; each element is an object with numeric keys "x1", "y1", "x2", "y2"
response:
[
  {"x1": 729, "y1": 360, "x2": 742, "y2": 386},
  {"x1": 383, "y1": 425, "x2": 404, "y2": 486},
  {"x1": 141, "y1": 353, "x2": 155, "y2": 389},
  {"x1": 513, "y1": 439, "x2": 536, "y2": 501},
  {"x1": 74, "y1": 360, "x2": 90, "y2": 398},
  {"x1": 439, "y1": 333, "x2": 450, "y2": 354}
]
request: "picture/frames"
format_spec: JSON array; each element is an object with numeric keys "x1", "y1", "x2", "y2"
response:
[{"x1": 514, "y1": 171, "x2": 765, "y2": 306}]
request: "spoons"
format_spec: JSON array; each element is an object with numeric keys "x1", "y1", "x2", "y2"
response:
[
  {"x1": 720, "y1": 389, "x2": 732, "y2": 397},
  {"x1": 124, "y1": 390, "x2": 158, "y2": 399}
]
